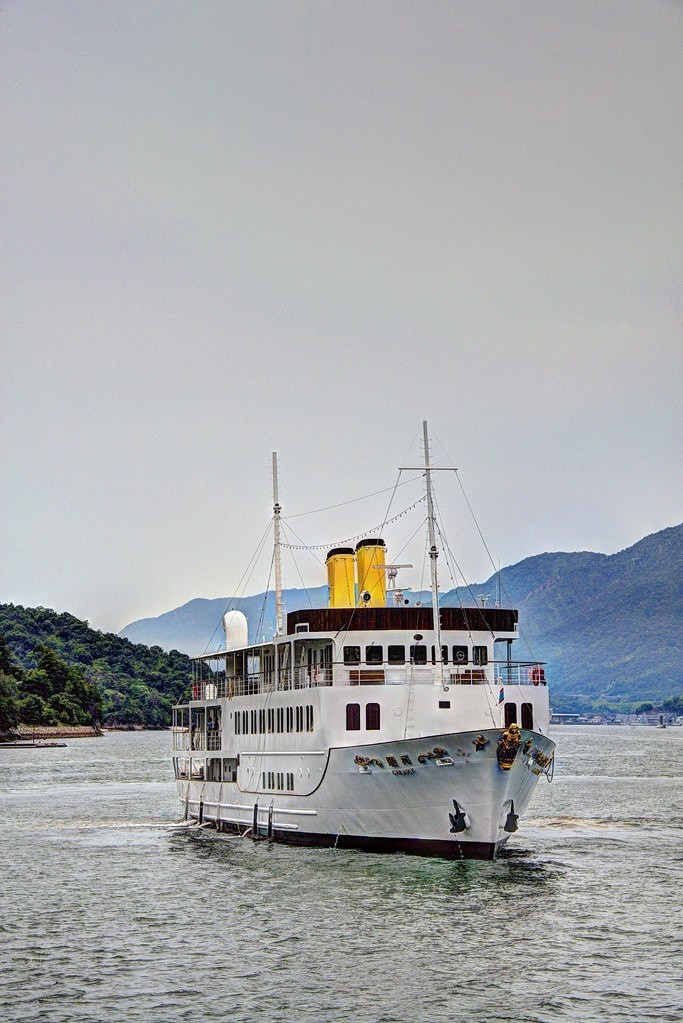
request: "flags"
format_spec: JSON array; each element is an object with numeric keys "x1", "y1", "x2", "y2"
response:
[{"x1": 495, "y1": 678, "x2": 504, "y2": 706}]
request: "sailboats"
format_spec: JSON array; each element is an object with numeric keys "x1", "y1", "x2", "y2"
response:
[{"x1": 171, "y1": 420, "x2": 556, "y2": 861}]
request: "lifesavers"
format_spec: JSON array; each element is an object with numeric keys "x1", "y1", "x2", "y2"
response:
[{"x1": 531, "y1": 667, "x2": 541, "y2": 686}]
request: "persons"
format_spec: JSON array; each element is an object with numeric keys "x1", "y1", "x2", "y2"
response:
[
  {"x1": 207, "y1": 717, "x2": 219, "y2": 751},
  {"x1": 185, "y1": 719, "x2": 195, "y2": 751}
]
